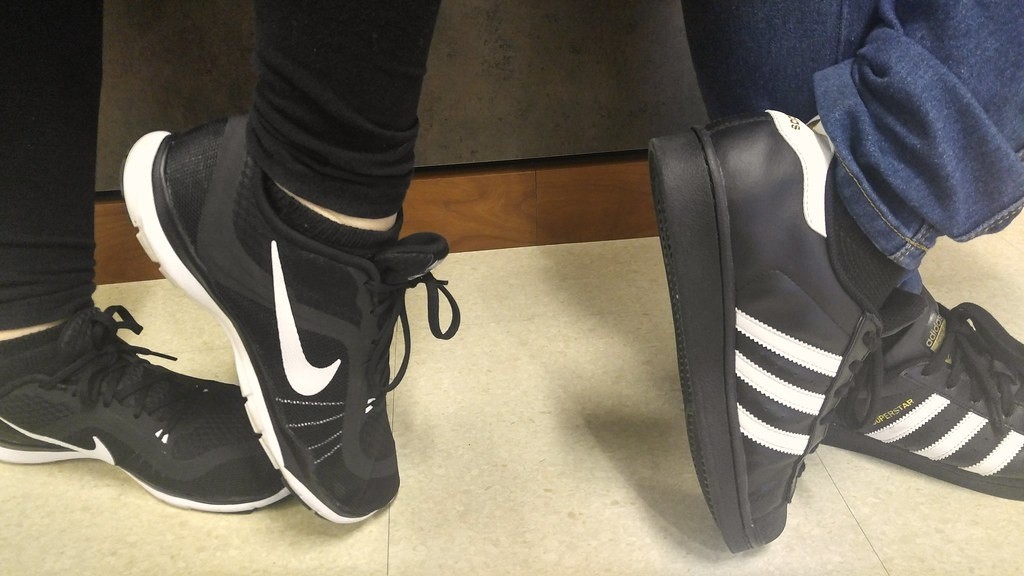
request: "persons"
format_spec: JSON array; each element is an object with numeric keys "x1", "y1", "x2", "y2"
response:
[
  {"x1": 0, "y1": 0, "x2": 461, "y2": 526},
  {"x1": 648, "y1": 0, "x2": 1024, "y2": 553}
]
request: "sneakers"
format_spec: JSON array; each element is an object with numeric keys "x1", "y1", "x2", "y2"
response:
[
  {"x1": 120, "y1": 117, "x2": 460, "y2": 521},
  {"x1": 0, "y1": 306, "x2": 291, "y2": 514},
  {"x1": 821, "y1": 287, "x2": 1024, "y2": 501},
  {"x1": 652, "y1": 108, "x2": 928, "y2": 555}
]
